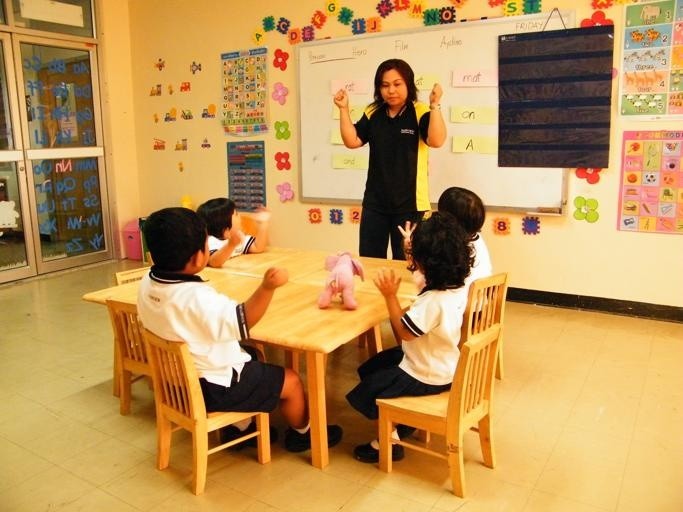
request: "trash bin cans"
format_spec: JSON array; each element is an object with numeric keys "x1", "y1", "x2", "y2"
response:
[{"x1": 121, "y1": 216, "x2": 144, "y2": 262}]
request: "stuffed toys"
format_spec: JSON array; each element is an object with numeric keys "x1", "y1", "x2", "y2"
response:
[{"x1": 316, "y1": 248, "x2": 365, "y2": 312}]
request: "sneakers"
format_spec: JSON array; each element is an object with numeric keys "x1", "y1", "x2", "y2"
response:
[
  {"x1": 285, "y1": 424, "x2": 342, "y2": 452},
  {"x1": 220, "y1": 420, "x2": 278, "y2": 451}
]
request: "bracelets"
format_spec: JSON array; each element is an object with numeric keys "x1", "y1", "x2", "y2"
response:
[{"x1": 429, "y1": 103, "x2": 441, "y2": 110}]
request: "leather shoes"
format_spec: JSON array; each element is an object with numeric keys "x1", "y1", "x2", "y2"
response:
[
  {"x1": 395, "y1": 423, "x2": 415, "y2": 439},
  {"x1": 353, "y1": 437, "x2": 404, "y2": 463}
]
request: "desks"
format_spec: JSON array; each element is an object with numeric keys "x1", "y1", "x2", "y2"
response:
[{"x1": 81, "y1": 249, "x2": 428, "y2": 470}]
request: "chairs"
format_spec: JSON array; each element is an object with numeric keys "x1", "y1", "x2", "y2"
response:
[
  {"x1": 144, "y1": 332, "x2": 273, "y2": 498},
  {"x1": 373, "y1": 323, "x2": 500, "y2": 497},
  {"x1": 105, "y1": 300, "x2": 154, "y2": 417},
  {"x1": 115, "y1": 266, "x2": 151, "y2": 286},
  {"x1": 459, "y1": 271, "x2": 513, "y2": 383}
]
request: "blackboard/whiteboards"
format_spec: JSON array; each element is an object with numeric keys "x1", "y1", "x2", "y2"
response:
[{"x1": 293, "y1": 10, "x2": 575, "y2": 218}]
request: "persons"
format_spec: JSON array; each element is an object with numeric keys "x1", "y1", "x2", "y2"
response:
[
  {"x1": 344, "y1": 214, "x2": 476, "y2": 464},
  {"x1": 136, "y1": 207, "x2": 344, "y2": 453},
  {"x1": 197, "y1": 197, "x2": 272, "y2": 267},
  {"x1": 396, "y1": 187, "x2": 492, "y2": 328},
  {"x1": 333, "y1": 59, "x2": 447, "y2": 261}
]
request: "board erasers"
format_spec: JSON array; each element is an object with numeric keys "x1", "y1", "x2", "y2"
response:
[{"x1": 539, "y1": 207, "x2": 562, "y2": 214}]
